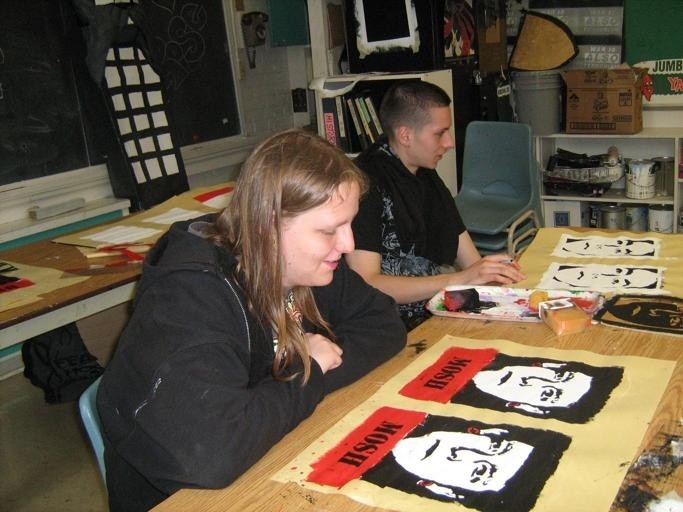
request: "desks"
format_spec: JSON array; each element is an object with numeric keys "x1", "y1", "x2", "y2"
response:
[
  {"x1": 147, "y1": 226, "x2": 683, "y2": 512},
  {"x1": 0, "y1": 178, "x2": 240, "y2": 349}
]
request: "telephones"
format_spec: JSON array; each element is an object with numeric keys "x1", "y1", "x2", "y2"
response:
[{"x1": 241, "y1": 11, "x2": 269, "y2": 47}]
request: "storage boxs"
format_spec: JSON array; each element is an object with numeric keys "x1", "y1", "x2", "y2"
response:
[
  {"x1": 558, "y1": 66, "x2": 650, "y2": 135},
  {"x1": 543, "y1": 200, "x2": 590, "y2": 228}
]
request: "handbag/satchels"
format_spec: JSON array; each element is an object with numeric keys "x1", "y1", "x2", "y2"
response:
[
  {"x1": 381, "y1": 252, "x2": 456, "y2": 321},
  {"x1": 22, "y1": 322, "x2": 106, "y2": 404}
]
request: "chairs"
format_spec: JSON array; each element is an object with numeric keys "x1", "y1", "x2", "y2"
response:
[
  {"x1": 78, "y1": 372, "x2": 107, "y2": 490},
  {"x1": 451, "y1": 120, "x2": 545, "y2": 264}
]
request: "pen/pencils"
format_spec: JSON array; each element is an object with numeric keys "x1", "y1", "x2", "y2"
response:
[{"x1": 499, "y1": 258, "x2": 514, "y2": 264}]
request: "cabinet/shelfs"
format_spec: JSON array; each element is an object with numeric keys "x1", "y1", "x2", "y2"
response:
[
  {"x1": 343, "y1": 0, "x2": 512, "y2": 192},
  {"x1": 314, "y1": 69, "x2": 458, "y2": 199},
  {"x1": 534, "y1": 125, "x2": 683, "y2": 234}
]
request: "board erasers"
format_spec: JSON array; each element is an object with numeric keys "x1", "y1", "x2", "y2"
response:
[{"x1": 29, "y1": 197, "x2": 86, "y2": 220}]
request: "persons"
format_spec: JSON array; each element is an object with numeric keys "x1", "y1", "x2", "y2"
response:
[
  {"x1": 554, "y1": 263, "x2": 658, "y2": 293},
  {"x1": 345, "y1": 79, "x2": 526, "y2": 326},
  {"x1": 563, "y1": 239, "x2": 655, "y2": 257},
  {"x1": 365, "y1": 412, "x2": 559, "y2": 510},
  {"x1": 461, "y1": 354, "x2": 605, "y2": 420},
  {"x1": 96, "y1": 128, "x2": 407, "y2": 512}
]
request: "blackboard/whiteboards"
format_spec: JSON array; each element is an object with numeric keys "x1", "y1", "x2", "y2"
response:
[{"x1": 0, "y1": 0, "x2": 254, "y2": 210}]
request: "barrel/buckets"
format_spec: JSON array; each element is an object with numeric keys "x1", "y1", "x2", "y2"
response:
[
  {"x1": 622, "y1": 204, "x2": 648, "y2": 232},
  {"x1": 601, "y1": 204, "x2": 626, "y2": 232},
  {"x1": 652, "y1": 155, "x2": 674, "y2": 197},
  {"x1": 514, "y1": 68, "x2": 568, "y2": 137},
  {"x1": 648, "y1": 204, "x2": 672, "y2": 234},
  {"x1": 624, "y1": 157, "x2": 655, "y2": 200}
]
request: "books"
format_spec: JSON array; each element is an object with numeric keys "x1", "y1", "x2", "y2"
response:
[{"x1": 321, "y1": 90, "x2": 384, "y2": 154}]
